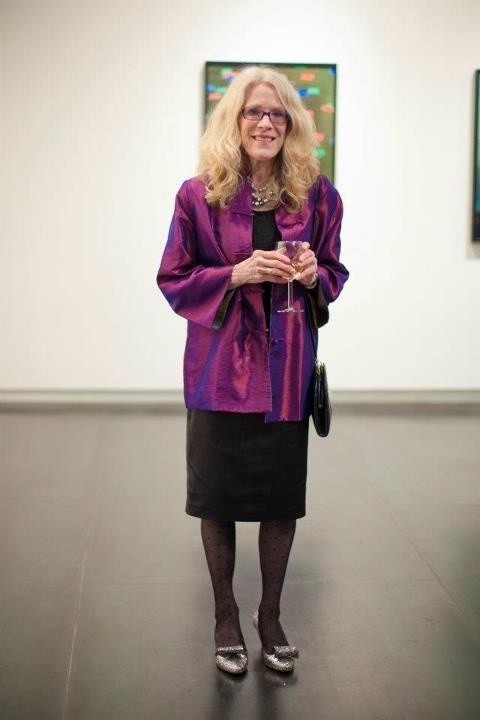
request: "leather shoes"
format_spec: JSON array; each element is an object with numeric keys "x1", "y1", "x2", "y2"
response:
[
  {"x1": 216, "y1": 643, "x2": 249, "y2": 676},
  {"x1": 252, "y1": 609, "x2": 299, "y2": 673}
]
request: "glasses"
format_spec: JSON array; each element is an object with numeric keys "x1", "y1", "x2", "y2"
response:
[{"x1": 239, "y1": 106, "x2": 291, "y2": 123}]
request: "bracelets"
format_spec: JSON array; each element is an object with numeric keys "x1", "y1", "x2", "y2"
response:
[{"x1": 303, "y1": 281, "x2": 318, "y2": 289}]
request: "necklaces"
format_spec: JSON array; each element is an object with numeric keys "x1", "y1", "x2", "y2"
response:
[{"x1": 248, "y1": 180, "x2": 275, "y2": 207}]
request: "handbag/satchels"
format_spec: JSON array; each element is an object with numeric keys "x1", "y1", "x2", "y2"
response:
[{"x1": 313, "y1": 359, "x2": 334, "y2": 438}]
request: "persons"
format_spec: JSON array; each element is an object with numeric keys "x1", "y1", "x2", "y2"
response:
[{"x1": 155, "y1": 62, "x2": 351, "y2": 676}]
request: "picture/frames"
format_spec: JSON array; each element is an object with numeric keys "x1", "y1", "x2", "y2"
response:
[{"x1": 202, "y1": 61, "x2": 337, "y2": 187}]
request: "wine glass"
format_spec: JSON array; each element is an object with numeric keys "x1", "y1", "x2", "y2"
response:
[{"x1": 275, "y1": 239, "x2": 309, "y2": 315}]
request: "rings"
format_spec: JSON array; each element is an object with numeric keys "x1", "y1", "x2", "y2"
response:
[{"x1": 268, "y1": 268, "x2": 273, "y2": 275}]
